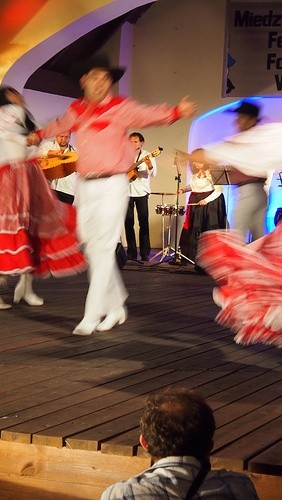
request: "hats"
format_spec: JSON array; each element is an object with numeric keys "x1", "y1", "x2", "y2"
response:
[
  {"x1": 228, "y1": 102, "x2": 259, "y2": 116},
  {"x1": 68, "y1": 55, "x2": 125, "y2": 84}
]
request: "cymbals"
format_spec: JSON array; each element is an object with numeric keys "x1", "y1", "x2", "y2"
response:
[{"x1": 149, "y1": 191, "x2": 176, "y2": 196}]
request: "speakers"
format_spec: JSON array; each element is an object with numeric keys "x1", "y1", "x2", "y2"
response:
[{"x1": 115, "y1": 243, "x2": 127, "y2": 269}]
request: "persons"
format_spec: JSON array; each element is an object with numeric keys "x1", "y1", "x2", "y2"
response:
[
  {"x1": 0, "y1": 53, "x2": 282, "y2": 351},
  {"x1": 100, "y1": 386, "x2": 259, "y2": 500}
]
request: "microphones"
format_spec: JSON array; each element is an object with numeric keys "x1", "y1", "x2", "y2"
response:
[{"x1": 173, "y1": 156, "x2": 176, "y2": 167}]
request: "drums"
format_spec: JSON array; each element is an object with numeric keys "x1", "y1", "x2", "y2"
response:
[
  {"x1": 171, "y1": 204, "x2": 186, "y2": 215},
  {"x1": 156, "y1": 204, "x2": 173, "y2": 216}
]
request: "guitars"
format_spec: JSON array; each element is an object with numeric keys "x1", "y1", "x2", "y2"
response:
[{"x1": 127, "y1": 146, "x2": 162, "y2": 182}]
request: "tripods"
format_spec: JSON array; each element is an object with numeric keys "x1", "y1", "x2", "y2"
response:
[{"x1": 148, "y1": 161, "x2": 195, "y2": 267}]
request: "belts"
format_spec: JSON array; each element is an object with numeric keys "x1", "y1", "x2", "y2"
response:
[
  {"x1": 238, "y1": 179, "x2": 263, "y2": 187},
  {"x1": 86, "y1": 174, "x2": 112, "y2": 180}
]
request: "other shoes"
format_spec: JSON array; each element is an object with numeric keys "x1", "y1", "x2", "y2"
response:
[
  {"x1": 72, "y1": 317, "x2": 100, "y2": 335},
  {"x1": 13, "y1": 273, "x2": 44, "y2": 305},
  {"x1": 96, "y1": 307, "x2": 126, "y2": 331},
  {"x1": 0, "y1": 297, "x2": 12, "y2": 310},
  {"x1": 140, "y1": 257, "x2": 149, "y2": 261}
]
